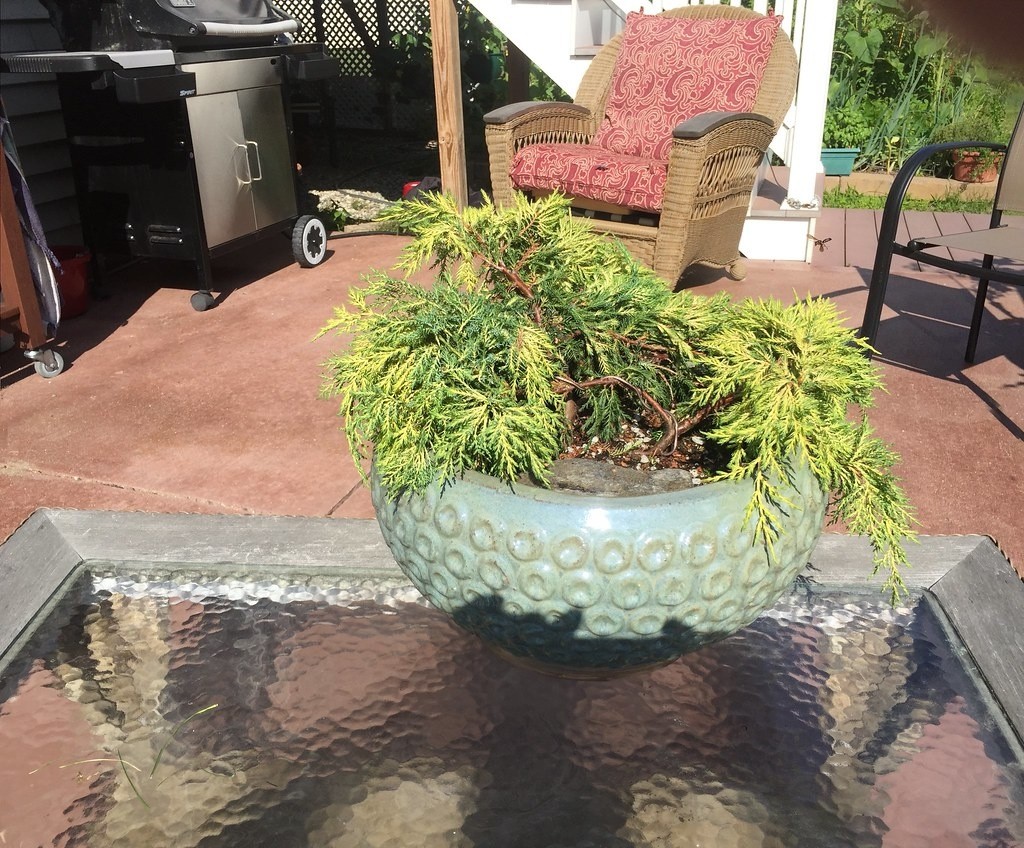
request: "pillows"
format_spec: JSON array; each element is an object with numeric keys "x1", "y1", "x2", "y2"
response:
[{"x1": 589, "y1": 11, "x2": 783, "y2": 158}]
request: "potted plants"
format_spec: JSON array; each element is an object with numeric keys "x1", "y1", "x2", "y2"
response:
[
  {"x1": 822, "y1": 107, "x2": 871, "y2": 176},
  {"x1": 311, "y1": 181, "x2": 924, "y2": 680},
  {"x1": 954, "y1": 85, "x2": 1009, "y2": 183}
]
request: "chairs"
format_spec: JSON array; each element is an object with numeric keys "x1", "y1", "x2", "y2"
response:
[
  {"x1": 484, "y1": 4, "x2": 800, "y2": 295},
  {"x1": 859, "y1": 99, "x2": 1024, "y2": 366}
]
